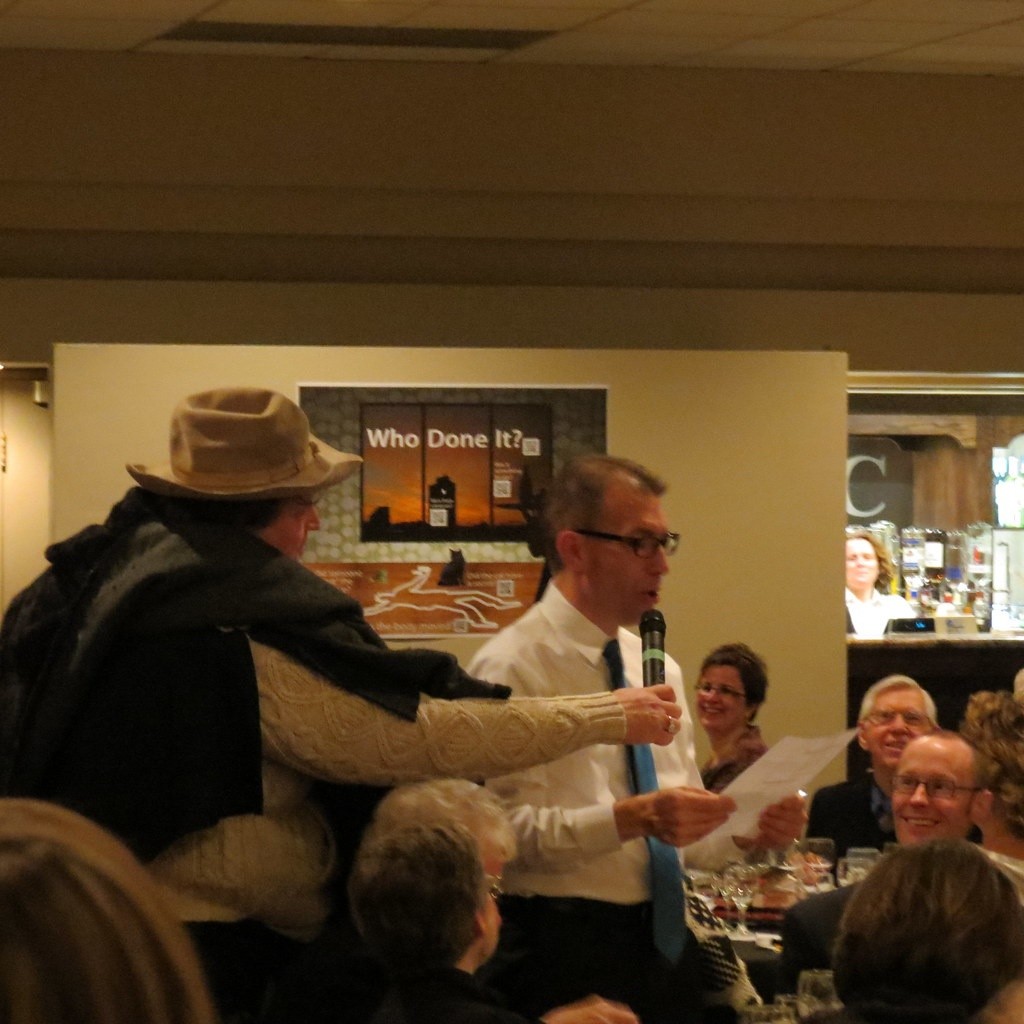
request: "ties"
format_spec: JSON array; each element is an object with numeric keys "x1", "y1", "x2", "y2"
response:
[{"x1": 602, "y1": 638, "x2": 688, "y2": 963}]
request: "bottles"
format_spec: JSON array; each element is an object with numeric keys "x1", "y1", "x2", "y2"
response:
[{"x1": 871, "y1": 521, "x2": 995, "y2": 624}]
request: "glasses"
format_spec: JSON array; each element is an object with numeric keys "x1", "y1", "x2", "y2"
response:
[
  {"x1": 891, "y1": 774, "x2": 979, "y2": 799},
  {"x1": 572, "y1": 530, "x2": 679, "y2": 558},
  {"x1": 864, "y1": 708, "x2": 931, "y2": 726},
  {"x1": 693, "y1": 681, "x2": 748, "y2": 697}
]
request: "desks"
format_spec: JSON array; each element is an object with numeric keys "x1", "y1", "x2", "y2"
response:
[{"x1": 696, "y1": 861, "x2": 838, "y2": 924}]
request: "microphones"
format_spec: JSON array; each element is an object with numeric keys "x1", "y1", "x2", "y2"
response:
[{"x1": 639, "y1": 608, "x2": 666, "y2": 688}]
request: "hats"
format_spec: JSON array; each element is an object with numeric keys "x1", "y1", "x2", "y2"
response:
[{"x1": 126, "y1": 387, "x2": 363, "y2": 500}]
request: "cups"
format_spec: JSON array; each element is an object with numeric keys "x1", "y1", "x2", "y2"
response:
[
  {"x1": 836, "y1": 843, "x2": 900, "y2": 886},
  {"x1": 741, "y1": 970, "x2": 843, "y2": 1024}
]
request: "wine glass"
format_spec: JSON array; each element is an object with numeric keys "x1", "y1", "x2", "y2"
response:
[
  {"x1": 723, "y1": 864, "x2": 758, "y2": 936},
  {"x1": 801, "y1": 837, "x2": 835, "y2": 895}
]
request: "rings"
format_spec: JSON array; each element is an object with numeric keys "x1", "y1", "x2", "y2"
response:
[{"x1": 667, "y1": 716, "x2": 675, "y2": 733}]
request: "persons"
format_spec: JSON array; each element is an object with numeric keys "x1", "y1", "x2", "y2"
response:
[
  {"x1": 464, "y1": 454, "x2": 807, "y2": 1024},
  {"x1": 693, "y1": 641, "x2": 770, "y2": 794},
  {"x1": 846, "y1": 532, "x2": 920, "y2": 636},
  {"x1": 262, "y1": 778, "x2": 641, "y2": 1024},
  {"x1": 766, "y1": 674, "x2": 1024, "y2": 1024},
  {"x1": 0, "y1": 388, "x2": 683, "y2": 1024}
]
